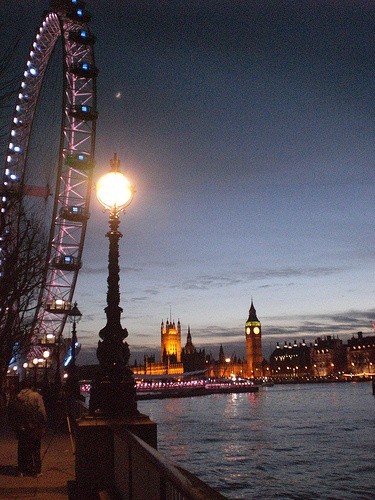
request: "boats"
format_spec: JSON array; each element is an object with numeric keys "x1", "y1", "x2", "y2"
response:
[{"x1": 262, "y1": 378, "x2": 275, "y2": 387}]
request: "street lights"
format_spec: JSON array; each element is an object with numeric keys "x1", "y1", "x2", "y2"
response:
[
  {"x1": 32, "y1": 357, "x2": 40, "y2": 389},
  {"x1": 67, "y1": 300, "x2": 83, "y2": 399},
  {"x1": 23, "y1": 362, "x2": 28, "y2": 387},
  {"x1": 42, "y1": 351, "x2": 50, "y2": 393},
  {"x1": 12, "y1": 365, "x2": 18, "y2": 376},
  {"x1": 88, "y1": 152, "x2": 143, "y2": 417}
]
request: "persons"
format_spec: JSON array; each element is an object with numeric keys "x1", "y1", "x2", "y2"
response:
[{"x1": 10, "y1": 378, "x2": 47, "y2": 477}]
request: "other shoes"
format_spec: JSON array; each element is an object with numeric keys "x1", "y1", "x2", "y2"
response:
[
  {"x1": 18, "y1": 471, "x2": 24, "y2": 478},
  {"x1": 36, "y1": 472, "x2": 43, "y2": 478}
]
